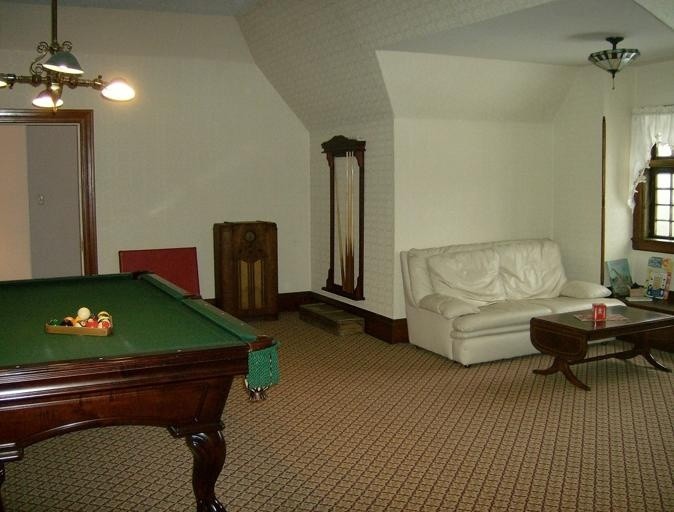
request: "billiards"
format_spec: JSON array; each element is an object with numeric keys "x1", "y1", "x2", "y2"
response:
[{"x1": 49, "y1": 307, "x2": 111, "y2": 329}]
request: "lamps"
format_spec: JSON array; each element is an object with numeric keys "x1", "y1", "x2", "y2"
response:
[
  {"x1": 0, "y1": 0, "x2": 135, "y2": 115},
  {"x1": 587, "y1": 36, "x2": 640, "y2": 91}
]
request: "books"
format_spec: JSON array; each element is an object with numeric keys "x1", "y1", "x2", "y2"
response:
[
  {"x1": 641, "y1": 256, "x2": 672, "y2": 300},
  {"x1": 605, "y1": 257, "x2": 633, "y2": 298}
]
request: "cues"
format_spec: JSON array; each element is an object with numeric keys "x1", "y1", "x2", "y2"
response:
[{"x1": 331, "y1": 149, "x2": 355, "y2": 295}]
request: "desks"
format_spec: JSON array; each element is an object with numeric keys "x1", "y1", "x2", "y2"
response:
[{"x1": 0, "y1": 270, "x2": 279, "y2": 511}]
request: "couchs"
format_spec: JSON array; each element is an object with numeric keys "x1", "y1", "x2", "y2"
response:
[{"x1": 400, "y1": 237, "x2": 626, "y2": 370}]
